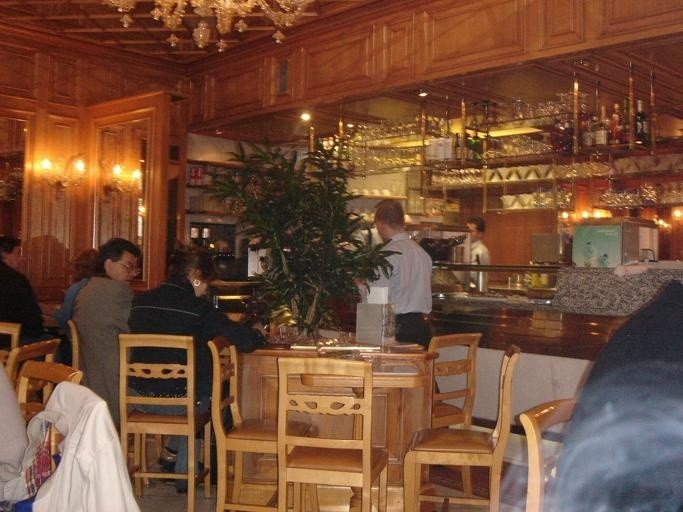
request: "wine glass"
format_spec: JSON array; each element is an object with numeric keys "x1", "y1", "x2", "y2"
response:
[
  {"x1": 598, "y1": 180, "x2": 683, "y2": 210},
  {"x1": 352, "y1": 113, "x2": 481, "y2": 184},
  {"x1": 497, "y1": 93, "x2": 573, "y2": 210}
]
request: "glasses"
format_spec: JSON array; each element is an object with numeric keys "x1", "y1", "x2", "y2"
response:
[{"x1": 117, "y1": 262, "x2": 142, "y2": 275}]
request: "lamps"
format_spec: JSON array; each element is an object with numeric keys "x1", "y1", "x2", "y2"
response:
[
  {"x1": 97, "y1": 1, "x2": 318, "y2": 54},
  {"x1": 39, "y1": 158, "x2": 87, "y2": 199},
  {"x1": 99, "y1": 159, "x2": 144, "y2": 195}
]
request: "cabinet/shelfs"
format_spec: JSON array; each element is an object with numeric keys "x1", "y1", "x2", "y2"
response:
[
  {"x1": 308, "y1": 63, "x2": 683, "y2": 217},
  {"x1": 183, "y1": 158, "x2": 249, "y2": 282}
]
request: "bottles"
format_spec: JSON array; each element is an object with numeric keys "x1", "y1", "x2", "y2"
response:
[
  {"x1": 189, "y1": 165, "x2": 242, "y2": 212},
  {"x1": 190, "y1": 228, "x2": 235, "y2": 277},
  {"x1": 581, "y1": 97, "x2": 650, "y2": 148},
  {"x1": 453, "y1": 131, "x2": 480, "y2": 161}
]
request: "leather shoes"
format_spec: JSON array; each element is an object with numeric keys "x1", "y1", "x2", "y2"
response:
[{"x1": 158, "y1": 447, "x2": 203, "y2": 493}]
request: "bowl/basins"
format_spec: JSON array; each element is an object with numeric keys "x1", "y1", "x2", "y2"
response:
[{"x1": 350, "y1": 188, "x2": 411, "y2": 224}]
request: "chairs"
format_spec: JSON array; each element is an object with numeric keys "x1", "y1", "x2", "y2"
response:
[
  {"x1": 277, "y1": 356, "x2": 388, "y2": 512},
  {"x1": 428, "y1": 333, "x2": 482, "y2": 498},
  {"x1": 519, "y1": 398, "x2": 577, "y2": 512},
  {"x1": 1, "y1": 322, "x2": 21, "y2": 368},
  {"x1": 15, "y1": 360, "x2": 85, "y2": 473},
  {"x1": 6, "y1": 338, "x2": 62, "y2": 404},
  {"x1": 403, "y1": 344, "x2": 521, "y2": 512},
  {"x1": 208, "y1": 333, "x2": 314, "y2": 512},
  {"x1": 68, "y1": 320, "x2": 80, "y2": 370},
  {"x1": 119, "y1": 334, "x2": 214, "y2": 512}
]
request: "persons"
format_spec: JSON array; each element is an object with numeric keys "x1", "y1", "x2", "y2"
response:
[
  {"x1": 36, "y1": 249, "x2": 99, "y2": 387},
  {"x1": 70, "y1": 237, "x2": 140, "y2": 430},
  {"x1": 549, "y1": 279, "x2": 683, "y2": 511},
  {"x1": 465, "y1": 217, "x2": 491, "y2": 284},
  {"x1": 0, "y1": 356, "x2": 28, "y2": 511},
  {"x1": 0, "y1": 235, "x2": 45, "y2": 370},
  {"x1": 127, "y1": 245, "x2": 267, "y2": 494},
  {"x1": 366, "y1": 198, "x2": 440, "y2": 393}
]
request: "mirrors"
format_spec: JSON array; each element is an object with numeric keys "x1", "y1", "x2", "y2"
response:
[{"x1": 88, "y1": 106, "x2": 157, "y2": 293}]
